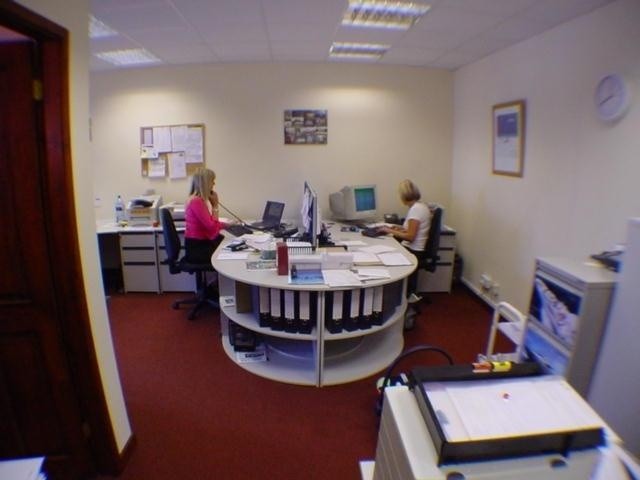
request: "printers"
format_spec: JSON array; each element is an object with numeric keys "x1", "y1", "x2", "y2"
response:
[
  {"x1": 159, "y1": 201, "x2": 187, "y2": 227},
  {"x1": 127, "y1": 195, "x2": 161, "y2": 225}
]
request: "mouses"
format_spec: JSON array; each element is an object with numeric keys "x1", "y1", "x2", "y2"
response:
[{"x1": 350, "y1": 227, "x2": 355, "y2": 231}]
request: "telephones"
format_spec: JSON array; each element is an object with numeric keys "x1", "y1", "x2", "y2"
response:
[{"x1": 384, "y1": 214, "x2": 400, "y2": 225}]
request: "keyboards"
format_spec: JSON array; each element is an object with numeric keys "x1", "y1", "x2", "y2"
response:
[
  {"x1": 225, "y1": 226, "x2": 252, "y2": 237},
  {"x1": 360, "y1": 226, "x2": 384, "y2": 237}
]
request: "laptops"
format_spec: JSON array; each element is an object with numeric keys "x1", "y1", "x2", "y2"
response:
[{"x1": 245, "y1": 201, "x2": 284, "y2": 230}]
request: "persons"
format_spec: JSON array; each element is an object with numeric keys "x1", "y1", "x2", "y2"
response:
[
  {"x1": 376, "y1": 179, "x2": 433, "y2": 265},
  {"x1": 184, "y1": 166, "x2": 240, "y2": 303}
]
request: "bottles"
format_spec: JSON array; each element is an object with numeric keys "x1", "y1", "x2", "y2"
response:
[
  {"x1": 115, "y1": 195, "x2": 125, "y2": 223},
  {"x1": 291, "y1": 265, "x2": 298, "y2": 279}
]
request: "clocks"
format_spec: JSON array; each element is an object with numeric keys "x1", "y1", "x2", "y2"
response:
[{"x1": 595, "y1": 74, "x2": 629, "y2": 122}]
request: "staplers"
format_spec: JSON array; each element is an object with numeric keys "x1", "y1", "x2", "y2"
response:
[{"x1": 232, "y1": 242, "x2": 249, "y2": 251}]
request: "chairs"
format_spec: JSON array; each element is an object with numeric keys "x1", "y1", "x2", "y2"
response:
[
  {"x1": 408, "y1": 206, "x2": 443, "y2": 316},
  {"x1": 159, "y1": 204, "x2": 220, "y2": 320}
]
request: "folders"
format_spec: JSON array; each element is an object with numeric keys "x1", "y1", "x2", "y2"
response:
[{"x1": 258, "y1": 286, "x2": 383, "y2": 334}]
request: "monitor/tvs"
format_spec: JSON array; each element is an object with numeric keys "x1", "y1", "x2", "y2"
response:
[{"x1": 329, "y1": 185, "x2": 377, "y2": 222}]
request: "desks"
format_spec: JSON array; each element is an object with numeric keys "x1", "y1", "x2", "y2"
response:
[{"x1": 211, "y1": 220, "x2": 418, "y2": 389}]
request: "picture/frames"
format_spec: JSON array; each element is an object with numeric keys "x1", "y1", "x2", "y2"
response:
[{"x1": 490, "y1": 98, "x2": 527, "y2": 177}]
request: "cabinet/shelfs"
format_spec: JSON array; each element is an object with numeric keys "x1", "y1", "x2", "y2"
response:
[
  {"x1": 515, "y1": 254, "x2": 615, "y2": 401},
  {"x1": 97, "y1": 220, "x2": 216, "y2": 295},
  {"x1": 419, "y1": 224, "x2": 456, "y2": 292},
  {"x1": 375, "y1": 357, "x2": 640, "y2": 480}
]
